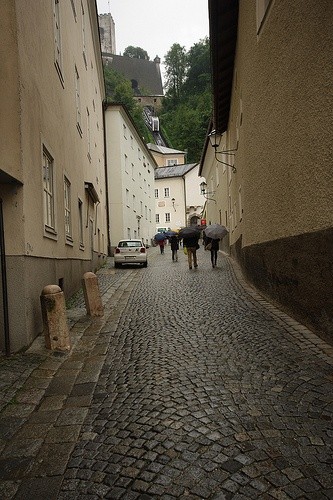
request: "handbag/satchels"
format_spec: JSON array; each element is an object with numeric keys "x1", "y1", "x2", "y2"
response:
[
  {"x1": 206, "y1": 243, "x2": 212, "y2": 250},
  {"x1": 184, "y1": 245, "x2": 188, "y2": 255}
]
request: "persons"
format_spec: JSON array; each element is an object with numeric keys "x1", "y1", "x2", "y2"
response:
[
  {"x1": 202, "y1": 230, "x2": 223, "y2": 268},
  {"x1": 155, "y1": 231, "x2": 200, "y2": 269}
]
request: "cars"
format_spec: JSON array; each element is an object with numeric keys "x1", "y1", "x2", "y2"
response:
[{"x1": 112, "y1": 239, "x2": 149, "y2": 268}]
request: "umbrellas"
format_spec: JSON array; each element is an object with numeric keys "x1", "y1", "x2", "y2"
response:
[
  {"x1": 165, "y1": 230, "x2": 178, "y2": 235},
  {"x1": 179, "y1": 226, "x2": 199, "y2": 237},
  {"x1": 205, "y1": 223, "x2": 229, "y2": 240},
  {"x1": 156, "y1": 232, "x2": 166, "y2": 241}
]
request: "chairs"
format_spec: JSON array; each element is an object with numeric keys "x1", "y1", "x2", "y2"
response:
[{"x1": 123, "y1": 243, "x2": 127, "y2": 247}]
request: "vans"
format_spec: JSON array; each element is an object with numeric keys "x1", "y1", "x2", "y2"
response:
[{"x1": 156, "y1": 227, "x2": 168, "y2": 234}]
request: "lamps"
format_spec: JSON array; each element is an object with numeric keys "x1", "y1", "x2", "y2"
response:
[
  {"x1": 199, "y1": 182, "x2": 219, "y2": 204},
  {"x1": 171, "y1": 198, "x2": 176, "y2": 212},
  {"x1": 207, "y1": 127, "x2": 239, "y2": 173}
]
